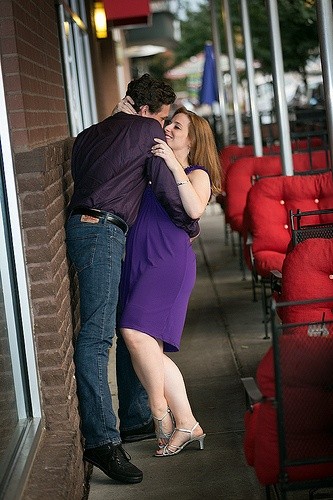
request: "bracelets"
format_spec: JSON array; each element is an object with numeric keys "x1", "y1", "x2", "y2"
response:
[{"x1": 177, "y1": 179, "x2": 190, "y2": 186}]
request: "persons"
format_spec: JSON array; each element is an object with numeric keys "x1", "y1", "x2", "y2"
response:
[
  {"x1": 112, "y1": 96, "x2": 223, "y2": 457},
  {"x1": 65, "y1": 74, "x2": 202, "y2": 481}
]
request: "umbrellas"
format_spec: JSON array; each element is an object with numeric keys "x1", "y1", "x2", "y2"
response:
[
  {"x1": 199, "y1": 40, "x2": 220, "y2": 114},
  {"x1": 166, "y1": 50, "x2": 261, "y2": 79}
]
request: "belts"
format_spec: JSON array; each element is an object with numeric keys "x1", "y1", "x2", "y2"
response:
[{"x1": 71, "y1": 209, "x2": 128, "y2": 238}]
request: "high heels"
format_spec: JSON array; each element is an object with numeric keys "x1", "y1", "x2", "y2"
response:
[
  {"x1": 155, "y1": 422, "x2": 206, "y2": 457},
  {"x1": 152, "y1": 405, "x2": 175, "y2": 449}
]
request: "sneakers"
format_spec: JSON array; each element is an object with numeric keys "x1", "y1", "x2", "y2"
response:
[
  {"x1": 83, "y1": 442, "x2": 143, "y2": 483},
  {"x1": 119, "y1": 419, "x2": 157, "y2": 444}
]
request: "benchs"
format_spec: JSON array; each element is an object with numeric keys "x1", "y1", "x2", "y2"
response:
[{"x1": 217, "y1": 142, "x2": 333, "y2": 500}]
